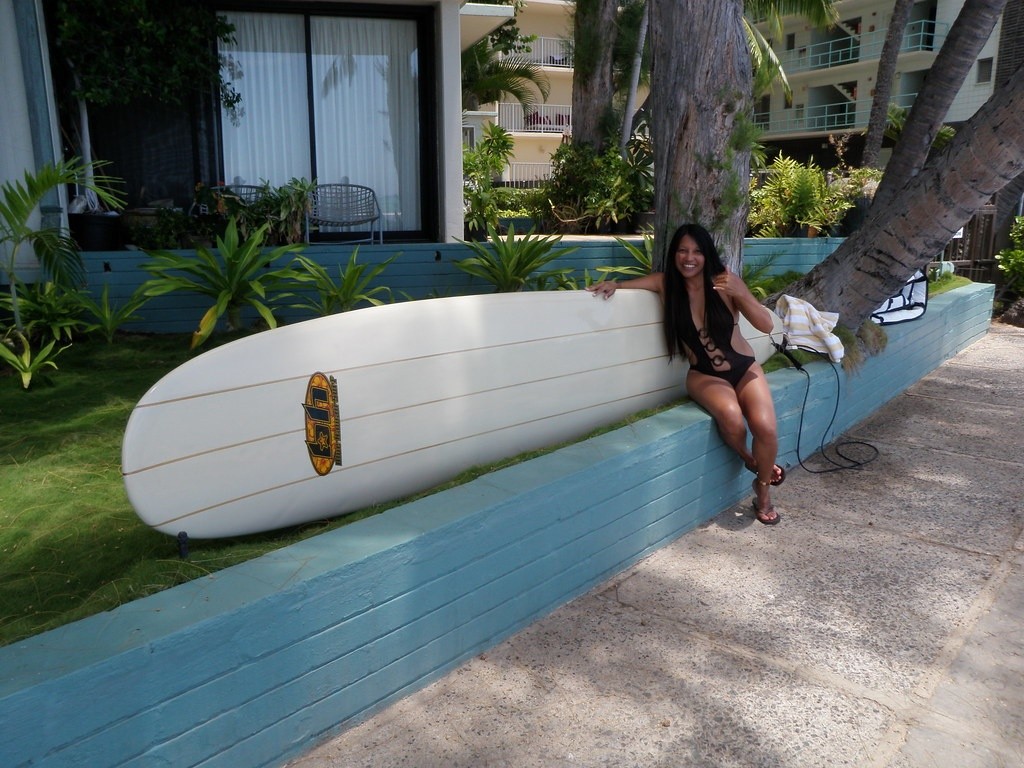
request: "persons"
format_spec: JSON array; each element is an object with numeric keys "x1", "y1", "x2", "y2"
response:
[{"x1": 584, "y1": 223, "x2": 785, "y2": 525}]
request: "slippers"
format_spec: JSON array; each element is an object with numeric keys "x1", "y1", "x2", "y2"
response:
[
  {"x1": 745, "y1": 462, "x2": 785, "y2": 486},
  {"x1": 753, "y1": 496, "x2": 781, "y2": 525}
]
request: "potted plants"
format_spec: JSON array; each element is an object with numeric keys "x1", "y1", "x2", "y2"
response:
[
  {"x1": 631, "y1": 188, "x2": 656, "y2": 235},
  {"x1": 463, "y1": 120, "x2": 516, "y2": 243},
  {"x1": 746, "y1": 147, "x2": 862, "y2": 238}
]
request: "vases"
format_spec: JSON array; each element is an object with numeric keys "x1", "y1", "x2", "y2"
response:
[{"x1": 68, "y1": 212, "x2": 127, "y2": 252}]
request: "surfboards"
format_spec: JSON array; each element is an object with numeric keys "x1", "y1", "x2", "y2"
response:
[{"x1": 121, "y1": 287, "x2": 791, "y2": 542}]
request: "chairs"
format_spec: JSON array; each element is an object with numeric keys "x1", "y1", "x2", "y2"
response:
[
  {"x1": 304, "y1": 183, "x2": 384, "y2": 245},
  {"x1": 189, "y1": 184, "x2": 279, "y2": 216}
]
